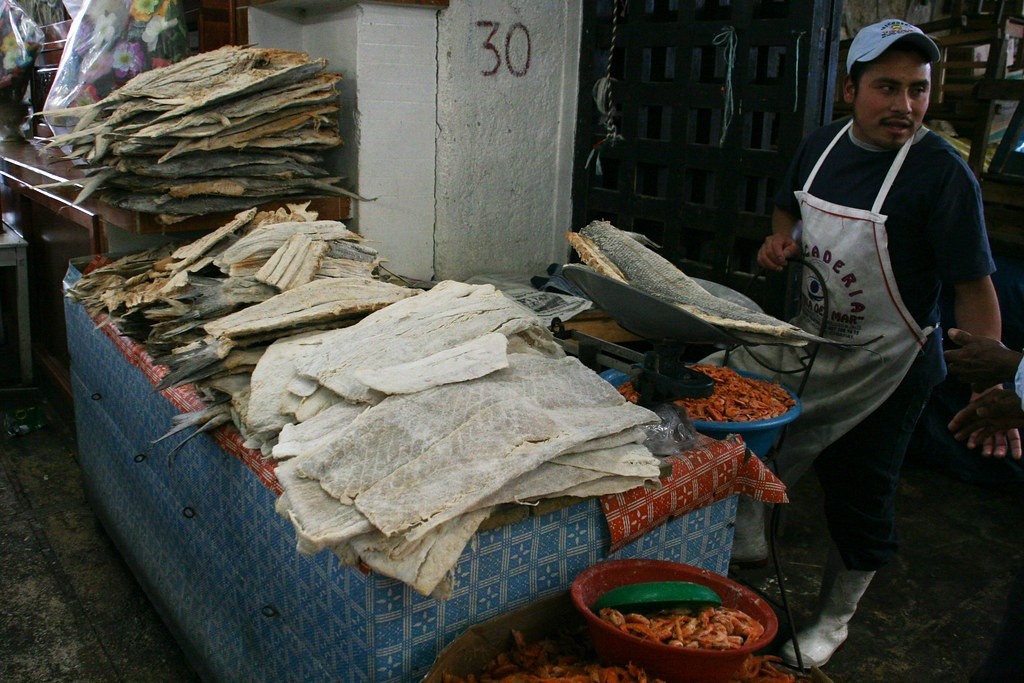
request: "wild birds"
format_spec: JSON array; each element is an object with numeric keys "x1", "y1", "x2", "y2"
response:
[{"x1": 67, "y1": 195, "x2": 441, "y2": 460}]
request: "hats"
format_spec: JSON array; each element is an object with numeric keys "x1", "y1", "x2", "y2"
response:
[{"x1": 846, "y1": 18, "x2": 941, "y2": 76}]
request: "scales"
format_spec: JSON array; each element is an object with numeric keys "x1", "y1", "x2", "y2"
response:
[{"x1": 550, "y1": 262, "x2": 810, "y2": 410}]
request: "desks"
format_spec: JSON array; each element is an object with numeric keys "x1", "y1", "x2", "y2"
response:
[{"x1": 0, "y1": 138, "x2": 349, "y2": 419}]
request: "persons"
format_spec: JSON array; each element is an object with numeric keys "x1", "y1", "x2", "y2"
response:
[
  {"x1": 697, "y1": 20, "x2": 1021, "y2": 669},
  {"x1": 944, "y1": 328, "x2": 1024, "y2": 450}
]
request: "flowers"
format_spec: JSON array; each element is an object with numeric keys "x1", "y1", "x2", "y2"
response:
[
  {"x1": 0, "y1": 0, "x2": 46, "y2": 74},
  {"x1": 41, "y1": 0, "x2": 190, "y2": 116}
]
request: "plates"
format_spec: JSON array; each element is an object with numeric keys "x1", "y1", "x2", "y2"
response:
[{"x1": 598, "y1": 581, "x2": 723, "y2": 615}]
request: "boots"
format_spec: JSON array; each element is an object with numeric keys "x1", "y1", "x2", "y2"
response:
[
  {"x1": 779, "y1": 538, "x2": 876, "y2": 669},
  {"x1": 728, "y1": 495, "x2": 769, "y2": 568}
]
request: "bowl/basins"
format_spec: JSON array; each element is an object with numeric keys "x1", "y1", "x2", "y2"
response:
[
  {"x1": 600, "y1": 363, "x2": 802, "y2": 457},
  {"x1": 571, "y1": 558, "x2": 779, "y2": 683}
]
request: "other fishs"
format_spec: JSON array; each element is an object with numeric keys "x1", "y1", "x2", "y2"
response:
[
  {"x1": 27, "y1": 42, "x2": 380, "y2": 216},
  {"x1": 566, "y1": 217, "x2": 885, "y2": 348}
]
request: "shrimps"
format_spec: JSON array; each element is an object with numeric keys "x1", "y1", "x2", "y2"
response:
[
  {"x1": 600, "y1": 603, "x2": 764, "y2": 650},
  {"x1": 439, "y1": 627, "x2": 648, "y2": 683},
  {"x1": 730, "y1": 654, "x2": 801, "y2": 683}
]
